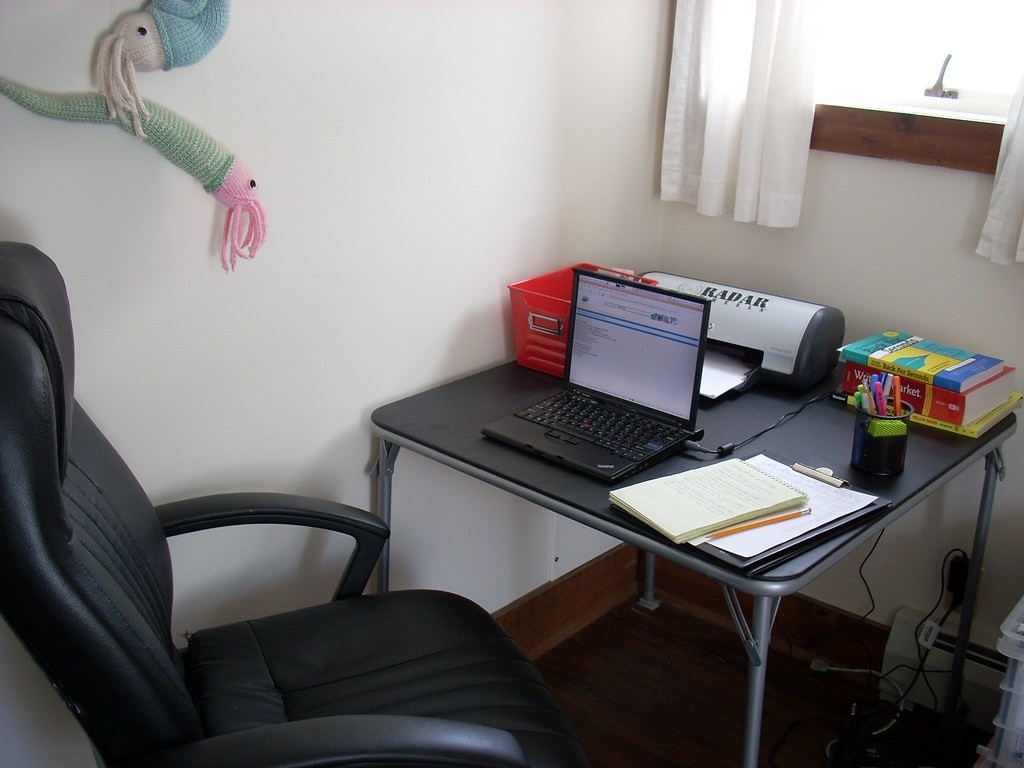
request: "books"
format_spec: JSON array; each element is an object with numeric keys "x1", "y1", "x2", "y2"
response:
[
  {"x1": 608, "y1": 457, "x2": 810, "y2": 545},
  {"x1": 832, "y1": 330, "x2": 1023, "y2": 438}
]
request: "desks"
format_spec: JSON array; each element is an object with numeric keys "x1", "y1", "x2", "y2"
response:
[{"x1": 371, "y1": 357, "x2": 1017, "y2": 768}]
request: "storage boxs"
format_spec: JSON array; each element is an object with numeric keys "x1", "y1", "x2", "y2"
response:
[{"x1": 507, "y1": 262, "x2": 659, "y2": 376}]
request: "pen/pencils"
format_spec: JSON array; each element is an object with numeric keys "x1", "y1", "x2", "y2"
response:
[
  {"x1": 704, "y1": 507, "x2": 812, "y2": 539},
  {"x1": 853, "y1": 372, "x2": 902, "y2": 474}
]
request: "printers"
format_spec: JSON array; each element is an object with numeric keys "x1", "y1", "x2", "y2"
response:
[{"x1": 633, "y1": 271, "x2": 845, "y2": 408}]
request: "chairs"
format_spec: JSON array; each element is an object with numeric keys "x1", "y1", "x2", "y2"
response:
[{"x1": 1, "y1": 240, "x2": 581, "y2": 768}]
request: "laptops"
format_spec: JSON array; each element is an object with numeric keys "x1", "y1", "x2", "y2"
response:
[{"x1": 482, "y1": 266, "x2": 711, "y2": 485}]
